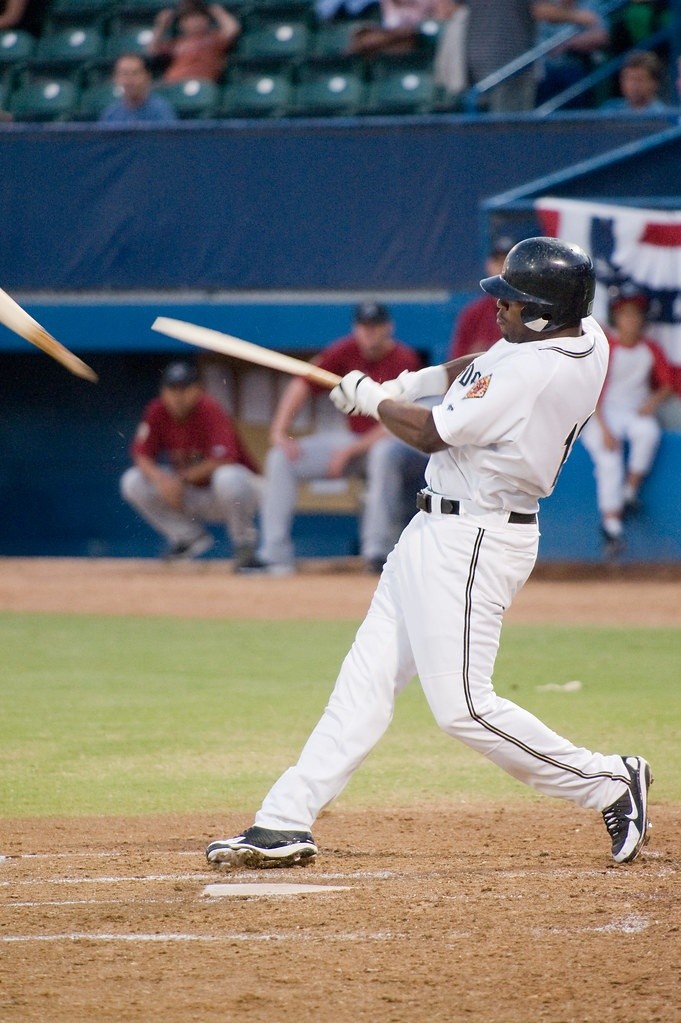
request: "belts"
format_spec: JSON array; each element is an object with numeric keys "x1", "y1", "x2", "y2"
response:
[{"x1": 416, "y1": 491, "x2": 537, "y2": 525}]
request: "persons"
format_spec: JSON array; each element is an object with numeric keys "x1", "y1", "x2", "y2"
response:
[
  {"x1": 147, "y1": 0, "x2": 242, "y2": 83},
  {"x1": 450, "y1": 236, "x2": 522, "y2": 360},
  {"x1": 238, "y1": 299, "x2": 419, "y2": 577},
  {"x1": 0, "y1": 0, "x2": 46, "y2": 37},
  {"x1": 347, "y1": 0, "x2": 610, "y2": 112},
  {"x1": 97, "y1": 53, "x2": 177, "y2": 123},
  {"x1": 597, "y1": 50, "x2": 672, "y2": 114},
  {"x1": 205, "y1": 236, "x2": 654, "y2": 872},
  {"x1": 579, "y1": 278, "x2": 676, "y2": 546},
  {"x1": 119, "y1": 361, "x2": 261, "y2": 574}
]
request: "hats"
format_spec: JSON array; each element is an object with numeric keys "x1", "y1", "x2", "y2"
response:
[
  {"x1": 163, "y1": 364, "x2": 197, "y2": 387},
  {"x1": 355, "y1": 302, "x2": 389, "y2": 326}
]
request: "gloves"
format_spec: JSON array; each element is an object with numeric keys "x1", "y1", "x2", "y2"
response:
[
  {"x1": 330, "y1": 369, "x2": 392, "y2": 420},
  {"x1": 378, "y1": 364, "x2": 449, "y2": 403}
]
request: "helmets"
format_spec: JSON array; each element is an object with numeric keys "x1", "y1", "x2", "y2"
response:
[{"x1": 480, "y1": 238, "x2": 597, "y2": 333}]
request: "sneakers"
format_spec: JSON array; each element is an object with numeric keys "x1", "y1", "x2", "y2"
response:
[
  {"x1": 602, "y1": 755, "x2": 654, "y2": 862},
  {"x1": 207, "y1": 826, "x2": 316, "y2": 868}
]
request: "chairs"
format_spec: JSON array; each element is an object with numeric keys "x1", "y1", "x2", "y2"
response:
[{"x1": 0, "y1": 2, "x2": 451, "y2": 122}]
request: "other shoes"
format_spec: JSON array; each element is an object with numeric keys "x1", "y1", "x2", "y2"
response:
[
  {"x1": 597, "y1": 497, "x2": 644, "y2": 548},
  {"x1": 166, "y1": 531, "x2": 213, "y2": 561},
  {"x1": 235, "y1": 558, "x2": 295, "y2": 575}
]
